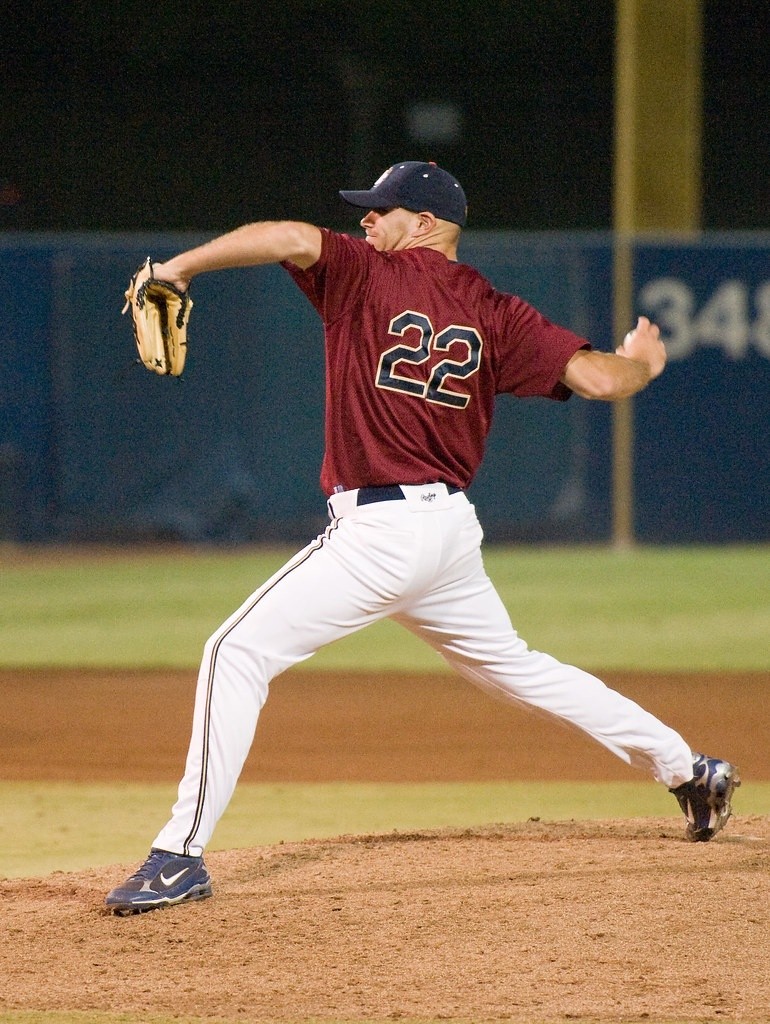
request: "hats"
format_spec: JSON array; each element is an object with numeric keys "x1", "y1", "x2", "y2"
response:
[{"x1": 336, "y1": 159, "x2": 468, "y2": 229}]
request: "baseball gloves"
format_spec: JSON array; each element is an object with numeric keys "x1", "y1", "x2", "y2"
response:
[{"x1": 124, "y1": 260, "x2": 194, "y2": 382}]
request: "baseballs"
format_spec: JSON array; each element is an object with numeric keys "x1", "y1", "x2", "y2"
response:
[{"x1": 623, "y1": 328, "x2": 638, "y2": 351}]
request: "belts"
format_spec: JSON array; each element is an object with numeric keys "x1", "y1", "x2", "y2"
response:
[{"x1": 329, "y1": 482, "x2": 464, "y2": 518}]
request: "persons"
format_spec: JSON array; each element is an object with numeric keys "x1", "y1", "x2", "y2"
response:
[{"x1": 103, "y1": 161, "x2": 739, "y2": 911}]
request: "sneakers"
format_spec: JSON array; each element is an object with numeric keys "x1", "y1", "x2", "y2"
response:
[
  {"x1": 106, "y1": 850, "x2": 215, "y2": 912},
  {"x1": 668, "y1": 747, "x2": 743, "y2": 843}
]
